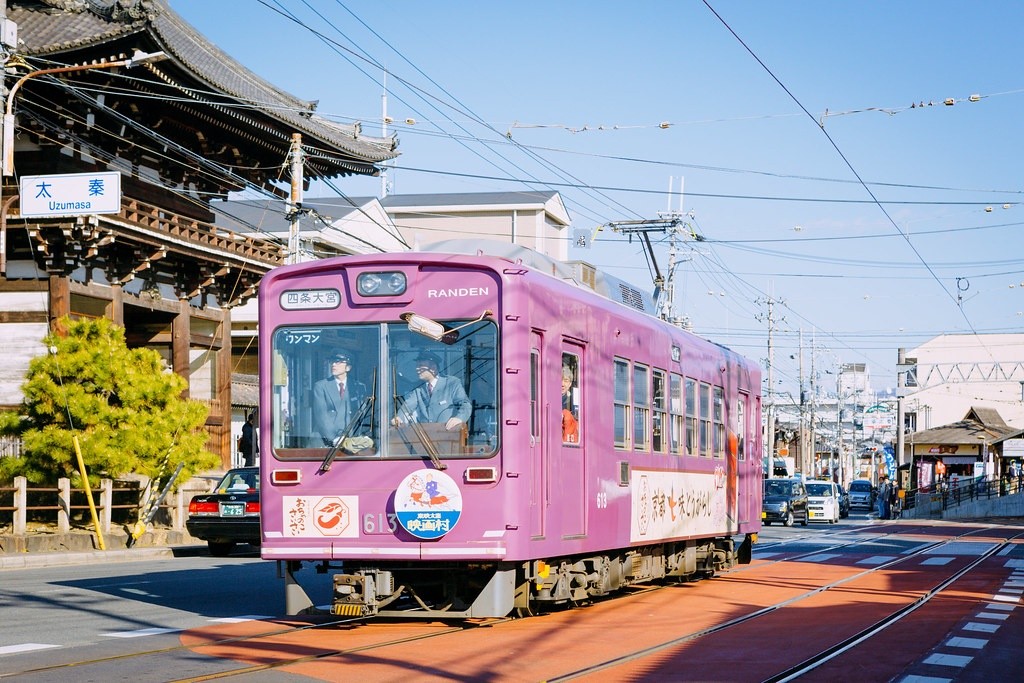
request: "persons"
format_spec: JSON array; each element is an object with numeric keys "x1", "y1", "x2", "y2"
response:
[
  {"x1": 562, "y1": 361, "x2": 576, "y2": 416},
  {"x1": 306, "y1": 347, "x2": 366, "y2": 448},
  {"x1": 390, "y1": 347, "x2": 472, "y2": 431},
  {"x1": 1019, "y1": 463, "x2": 1024, "y2": 490},
  {"x1": 238, "y1": 414, "x2": 259, "y2": 467},
  {"x1": 1009, "y1": 461, "x2": 1019, "y2": 494},
  {"x1": 876, "y1": 476, "x2": 897, "y2": 520}
]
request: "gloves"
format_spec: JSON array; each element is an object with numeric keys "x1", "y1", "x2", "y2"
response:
[{"x1": 332, "y1": 436, "x2": 348, "y2": 448}]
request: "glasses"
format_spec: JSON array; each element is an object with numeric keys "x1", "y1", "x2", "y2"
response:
[{"x1": 329, "y1": 358, "x2": 347, "y2": 364}]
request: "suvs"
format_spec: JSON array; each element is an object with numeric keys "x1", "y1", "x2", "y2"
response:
[{"x1": 762, "y1": 477, "x2": 810, "y2": 527}]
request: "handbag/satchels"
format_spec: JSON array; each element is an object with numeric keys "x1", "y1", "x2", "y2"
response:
[{"x1": 239, "y1": 440, "x2": 244, "y2": 452}]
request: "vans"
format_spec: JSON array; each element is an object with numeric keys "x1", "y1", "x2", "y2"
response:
[
  {"x1": 847, "y1": 481, "x2": 875, "y2": 510},
  {"x1": 804, "y1": 479, "x2": 841, "y2": 524}
]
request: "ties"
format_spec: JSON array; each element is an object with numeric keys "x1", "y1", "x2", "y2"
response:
[
  {"x1": 427, "y1": 383, "x2": 433, "y2": 398},
  {"x1": 339, "y1": 383, "x2": 345, "y2": 398}
]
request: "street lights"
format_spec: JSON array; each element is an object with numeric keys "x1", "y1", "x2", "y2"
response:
[{"x1": 1, "y1": 48, "x2": 172, "y2": 286}]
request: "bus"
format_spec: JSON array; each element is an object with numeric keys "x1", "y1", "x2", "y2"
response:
[{"x1": 260, "y1": 251, "x2": 762, "y2": 620}]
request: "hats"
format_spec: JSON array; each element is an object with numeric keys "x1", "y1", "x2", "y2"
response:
[
  {"x1": 328, "y1": 347, "x2": 353, "y2": 363},
  {"x1": 248, "y1": 414, "x2": 253, "y2": 419},
  {"x1": 412, "y1": 348, "x2": 444, "y2": 364}
]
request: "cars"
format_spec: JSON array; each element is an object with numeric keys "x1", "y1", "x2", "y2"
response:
[
  {"x1": 186, "y1": 468, "x2": 263, "y2": 552},
  {"x1": 837, "y1": 484, "x2": 850, "y2": 518}
]
